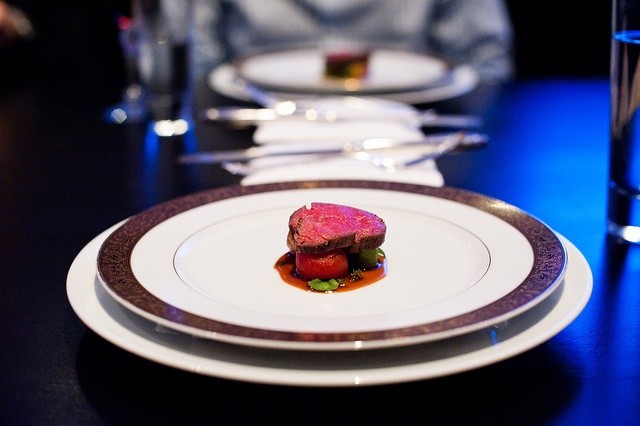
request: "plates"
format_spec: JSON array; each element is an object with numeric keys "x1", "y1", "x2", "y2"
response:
[
  {"x1": 206, "y1": 54, "x2": 481, "y2": 105},
  {"x1": 64, "y1": 211, "x2": 595, "y2": 389},
  {"x1": 234, "y1": 44, "x2": 456, "y2": 95},
  {"x1": 94, "y1": 180, "x2": 569, "y2": 355}
]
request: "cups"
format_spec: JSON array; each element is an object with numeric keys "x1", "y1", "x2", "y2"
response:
[
  {"x1": 128, "y1": 1, "x2": 191, "y2": 121},
  {"x1": 606, "y1": 0, "x2": 640, "y2": 245}
]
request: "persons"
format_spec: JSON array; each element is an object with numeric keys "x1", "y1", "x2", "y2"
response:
[{"x1": 185, "y1": 0, "x2": 519, "y2": 112}]
